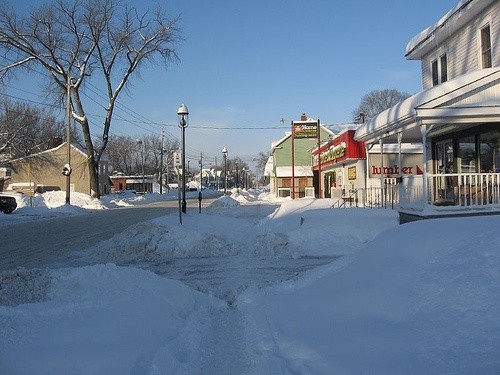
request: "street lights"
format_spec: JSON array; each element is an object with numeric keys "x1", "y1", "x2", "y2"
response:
[
  {"x1": 221, "y1": 146, "x2": 245, "y2": 189},
  {"x1": 176, "y1": 103, "x2": 190, "y2": 214}
]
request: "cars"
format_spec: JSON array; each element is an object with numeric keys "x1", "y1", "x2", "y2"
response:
[{"x1": 0, "y1": 196, "x2": 18, "y2": 214}]
